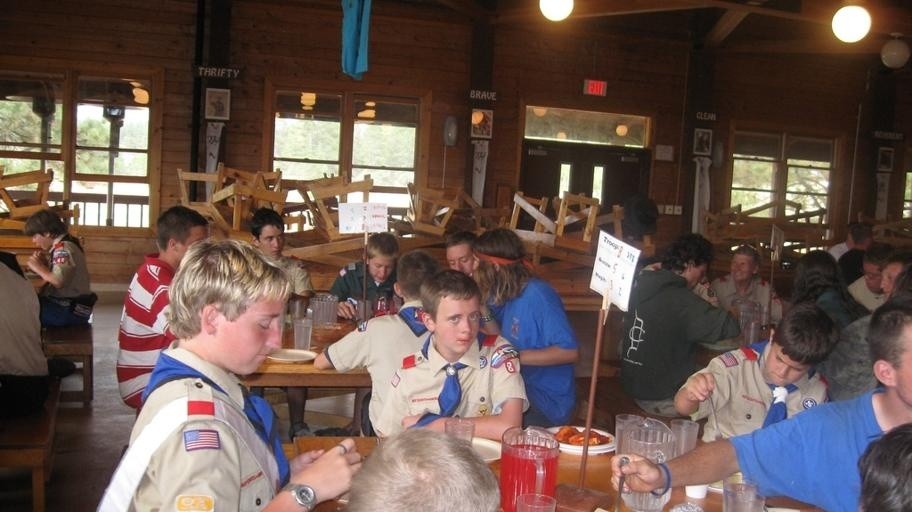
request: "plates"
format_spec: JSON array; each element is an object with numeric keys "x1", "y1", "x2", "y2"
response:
[
  {"x1": 707, "y1": 472, "x2": 744, "y2": 494},
  {"x1": 268, "y1": 349, "x2": 318, "y2": 364},
  {"x1": 472, "y1": 437, "x2": 503, "y2": 464},
  {"x1": 544, "y1": 426, "x2": 616, "y2": 456}
]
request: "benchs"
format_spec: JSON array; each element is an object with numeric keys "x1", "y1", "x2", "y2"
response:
[
  {"x1": 177, "y1": 162, "x2": 289, "y2": 246},
  {"x1": 510, "y1": 190, "x2": 623, "y2": 253},
  {"x1": 1, "y1": 379, "x2": 60, "y2": 512},
  {"x1": 43, "y1": 313, "x2": 94, "y2": 416},
  {"x1": 296, "y1": 174, "x2": 373, "y2": 242},
  {"x1": 0, "y1": 165, "x2": 54, "y2": 219},
  {"x1": 404, "y1": 183, "x2": 465, "y2": 235}
]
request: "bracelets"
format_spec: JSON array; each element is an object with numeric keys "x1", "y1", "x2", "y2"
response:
[
  {"x1": 481, "y1": 312, "x2": 495, "y2": 322},
  {"x1": 648, "y1": 463, "x2": 671, "y2": 496}
]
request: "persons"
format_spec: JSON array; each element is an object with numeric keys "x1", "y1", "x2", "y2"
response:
[
  {"x1": 837, "y1": 223, "x2": 874, "y2": 286},
  {"x1": 857, "y1": 425, "x2": 912, "y2": 512},
  {"x1": 712, "y1": 245, "x2": 782, "y2": 328},
  {"x1": 880, "y1": 252, "x2": 911, "y2": 298},
  {"x1": 793, "y1": 252, "x2": 873, "y2": 335},
  {"x1": 633, "y1": 234, "x2": 720, "y2": 308},
  {"x1": 25, "y1": 210, "x2": 98, "y2": 328},
  {"x1": 845, "y1": 242, "x2": 898, "y2": 310},
  {"x1": 827, "y1": 222, "x2": 858, "y2": 263},
  {"x1": 622, "y1": 239, "x2": 741, "y2": 417},
  {"x1": 375, "y1": 269, "x2": 529, "y2": 442},
  {"x1": 473, "y1": 227, "x2": 580, "y2": 428},
  {"x1": 611, "y1": 304, "x2": 912, "y2": 511},
  {"x1": 674, "y1": 303, "x2": 841, "y2": 443},
  {"x1": 127, "y1": 241, "x2": 366, "y2": 511},
  {"x1": 328, "y1": 233, "x2": 400, "y2": 434},
  {"x1": 314, "y1": 250, "x2": 438, "y2": 436},
  {"x1": 828, "y1": 266, "x2": 912, "y2": 403},
  {"x1": 446, "y1": 231, "x2": 473, "y2": 277},
  {"x1": 249, "y1": 207, "x2": 316, "y2": 442},
  {"x1": 116, "y1": 205, "x2": 207, "y2": 410},
  {"x1": 848, "y1": 208, "x2": 873, "y2": 233},
  {"x1": 0, "y1": 251, "x2": 51, "y2": 417}
]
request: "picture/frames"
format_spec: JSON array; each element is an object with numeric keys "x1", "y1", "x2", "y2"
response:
[
  {"x1": 692, "y1": 126, "x2": 715, "y2": 157},
  {"x1": 875, "y1": 145, "x2": 897, "y2": 174},
  {"x1": 202, "y1": 86, "x2": 233, "y2": 122},
  {"x1": 466, "y1": 105, "x2": 496, "y2": 141}
]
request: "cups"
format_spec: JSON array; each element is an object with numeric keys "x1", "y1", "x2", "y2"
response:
[
  {"x1": 723, "y1": 484, "x2": 766, "y2": 512},
  {"x1": 320, "y1": 295, "x2": 338, "y2": 325},
  {"x1": 670, "y1": 420, "x2": 699, "y2": 458},
  {"x1": 499, "y1": 426, "x2": 560, "y2": 511},
  {"x1": 357, "y1": 301, "x2": 373, "y2": 321},
  {"x1": 309, "y1": 297, "x2": 330, "y2": 331},
  {"x1": 685, "y1": 482, "x2": 708, "y2": 499},
  {"x1": 738, "y1": 303, "x2": 756, "y2": 337},
  {"x1": 517, "y1": 494, "x2": 557, "y2": 512},
  {"x1": 615, "y1": 415, "x2": 672, "y2": 512},
  {"x1": 293, "y1": 318, "x2": 313, "y2": 351},
  {"x1": 291, "y1": 299, "x2": 306, "y2": 324},
  {"x1": 744, "y1": 319, "x2": 761, "y2": 347}
]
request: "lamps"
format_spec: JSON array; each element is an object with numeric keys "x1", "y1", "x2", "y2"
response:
[
  {"x1": 540, "y1": 0, "x2": 574, "y2": 21},
  {"x1": 830, "y1": 5, "x2": 871, "y2": 43},
  {"x1": 881, "y1": 32, "x2": 911, "y2": 69}
]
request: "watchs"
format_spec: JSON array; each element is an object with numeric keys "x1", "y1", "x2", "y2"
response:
[{"x1": 283, "y1": 484, "x2": 319, "y2": 510}]
